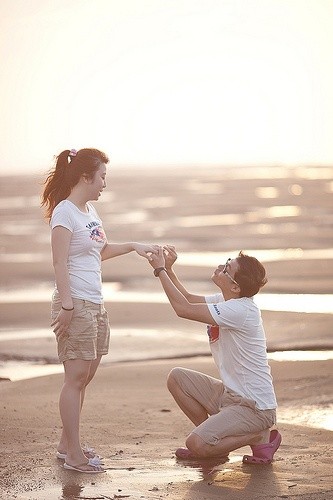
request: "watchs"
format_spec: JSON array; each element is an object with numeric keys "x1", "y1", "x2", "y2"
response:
[{"x1": 153, "y1": 267, "x2": 168, "y2": 277}]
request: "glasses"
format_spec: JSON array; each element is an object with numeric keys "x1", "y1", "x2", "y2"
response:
[{"x1": 223, "y1": 258, "x2": 239, "y2": 286}]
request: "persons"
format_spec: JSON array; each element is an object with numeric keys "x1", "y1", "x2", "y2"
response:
[
  {"x1": 148, "y1": 244, "x2": 282, "y2": 465},
  {"x1": 41, "y1": 149, "x2": 164, "y2": 475}
]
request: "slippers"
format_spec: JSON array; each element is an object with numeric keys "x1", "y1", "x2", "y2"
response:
[
  {"x1": 176, "y1": 447, "x2": 229, "y2": 458},
  {"x1": 57, "y1": 445, "x2": 96, "y2": 459},
  {"x1": 63, "y1": 456, "x2": 104, "y2": 473},
  {"x1": 243, "y1": 429, "x2": 282, "y2": 464}
]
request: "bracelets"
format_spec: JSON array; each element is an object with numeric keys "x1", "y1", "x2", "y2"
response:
[{"x1": 62, "y1": 306, "x2": 75, "y2": 312}]
